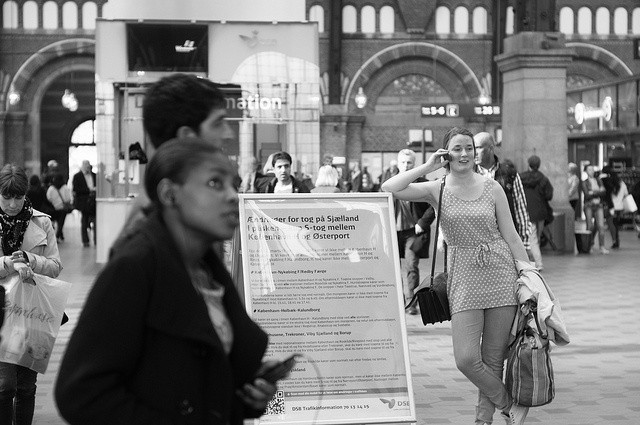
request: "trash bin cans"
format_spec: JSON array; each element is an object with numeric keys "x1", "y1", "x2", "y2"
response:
[{"x1": 574, "y1": 229, "x2": 594, "y2": 254}]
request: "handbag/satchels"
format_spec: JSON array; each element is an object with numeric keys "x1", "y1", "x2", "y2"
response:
[
  {"x1": 507, "y1": 301, "x2": 555, "y2": 406},
  {"x1": 404, "y1": 271, "x2": 451, "y2": 326},
  {"x1": 1, "y1": 272, "x2": 72, "y2": 374},
  {"x1": 623, "y1": 194, "x2": 638, "y2": 214}
]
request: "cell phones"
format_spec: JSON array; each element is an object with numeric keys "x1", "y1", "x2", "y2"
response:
[
  {"x1": 260, "y1": 357, "x2": 294, "y2": 382},
  {"x1": 442, "y1": 151, "x2": 452, "y2": 163}
]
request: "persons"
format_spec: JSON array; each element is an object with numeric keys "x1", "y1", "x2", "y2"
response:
[
  {"x1": 383, "y1": 159, "x2": 398, "y2": 182},
  {"x1": 346, "y1": 161, "x2": 362, "y2": 193},
  {"x1": 358, "y1": 173, "x2": 377, "y2": 193},
  {"x1": 47, "y1": 175, "x2": 73, "y2": 243},
  {"x1": 74, "y1": 160, "x2": 98, "y2": 245},
  {"x1": 43, "y1": 160, "x2": 60, "y2": 184},
  {"x1": 580, "y1": 163, "x2": 610, "y2": 254},
  {"x1": 310, "y1": 165, "x2": 340, "y2": 192},
  {"x1": 109, "y1": 74, "x2": 235, "y2": 266},
  {"x1": 55, "y1": 138, "x2": 281, "y2": 424},
  {"x1": 0, "y1": 162, "x2": 63, "y2": 424},
  {"x1": 380, "y1": 126, "x2": 537, "y2": 425},
  {"x1": 472, "y1": 131, "x2": 535, "y2": 263},
  {"x1": 519, "y1": 154, "x2": 554, "y2": 272},
  {"x1": 387, "y1": 146, "x2": 436, "y2": 315},
  {"x1": 26, "y1": 174, "x2": 55, "y2": 220},
  {"x1": 322, "y1": 155, "x2": 336, "y2": 167},
  {"x1": 603, "y1": 166, "x2": 630, "y2": 249},
  {"x1": 255, "y1": 150, "x2": 304, "y2": 192},
  {"x1": 566, "y1": 162, "x2": 581, "y2": 214}
]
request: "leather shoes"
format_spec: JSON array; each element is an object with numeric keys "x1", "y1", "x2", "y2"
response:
[{"x1": 405, "y1": 306, "x2": 418, "y2": 315}]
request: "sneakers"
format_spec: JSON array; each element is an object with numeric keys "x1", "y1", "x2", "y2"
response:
[
  {"x1": 601, "y1": 246, "x2": 610, "y2": 254},
  {"x1": 500, "y1": 405, "x2": 530, "y2": 425},
  {"x1": 611, "y1": 242, "x2": 619, "y2": 248}
]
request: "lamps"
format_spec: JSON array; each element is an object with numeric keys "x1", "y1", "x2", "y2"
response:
[
  {"x1": 60, "y1": 0, "x2": 78, "y2": 112},
  {"x1": 352, "y1": 0, "x2": 370, "y2": 110},
  {"x1": 478, "y1": 0, "x2": 490, "y2": 106},
  {"x1": 9, "y1": 0, "x2": 21, "y2": 106}
]
